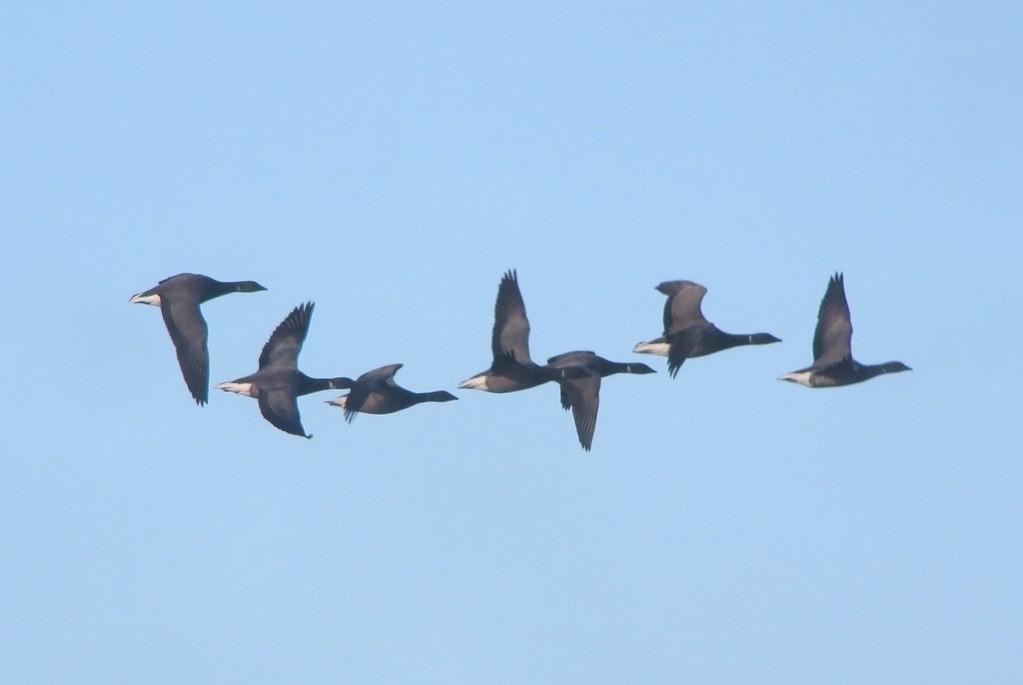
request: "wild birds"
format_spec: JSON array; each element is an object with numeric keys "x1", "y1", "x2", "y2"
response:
[
  {"x1": 208, "y1": 300, "x2": 356, "y2": 440},
  {"x1": 130, "y1": 273, "x2": 270, "y2": 407},
  {"x1": 540, "y1": 350, "x2": 657, "y2": 454},
  {"x1": 778, "y1": 272, "x2": 912, "y2": 388},
  {"x1": 456, "y1": 267, "x2": 593, "y2": 393},
  {"x1": 632, "y1": 280, "x2": 782, "y2": 379},
  {"x1": 325, "y1": 364, "x2": 460, "y2": 426}
]
